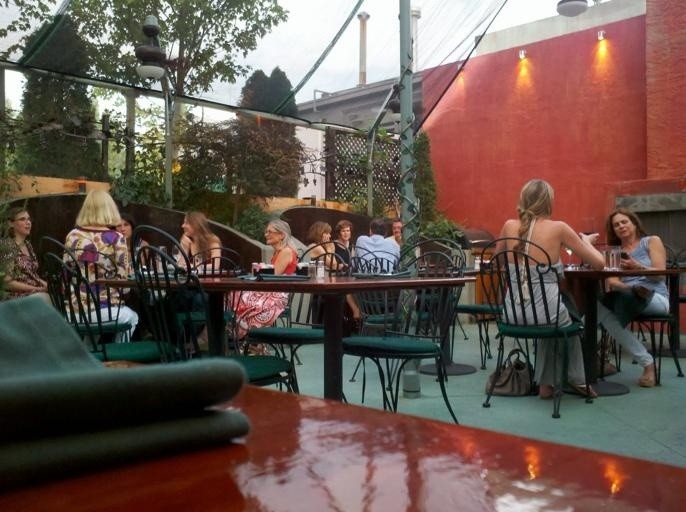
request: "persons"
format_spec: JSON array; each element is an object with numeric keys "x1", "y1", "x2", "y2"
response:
[
  {"x1": 354, "y1": 218, "x2": 400, "y2": 277},
  {"x1": 597, "y1": 208, "x2": 672, "y2": 387},
  {"x1": 116, "y1": 211, "x2": 149, "y2": 275},
  {"x1": 60, "y1": 188, "x2": 138, "y2": 344},
  {"x1": 305, "y1": 221, "x2": 366, "y2": 321},
  {"x1": 185, "y1": 220, "x2": 298, "y2": 352},
  {"x1": 1, "y1": 206, "x2": 48, "y2": 299},
  {"x1": 392, "y1": 214, "x2": 419, "y2": 262},
  {"x1": 493, "y1": 178, "x2": 604, "y2": 398},
  {"x1": 330, "y1": 220, "x2": 353, "y2": 262},
  {"x1": 176, "y1": 210, "x2": 221, "y2": 271}
]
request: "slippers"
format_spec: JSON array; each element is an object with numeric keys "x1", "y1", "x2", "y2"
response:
[
  {"x1": 539, "y1": 386, "x2": 564, "y2": 400},
  {"x1": 566, "y1": 379, "x2": 599, "y2": 399}
]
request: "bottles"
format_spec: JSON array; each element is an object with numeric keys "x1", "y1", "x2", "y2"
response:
[
  {"x1": 316, "y1": 260, "x2": 325, "y2": 278},
  {"x1": 474, "y1": 257, "x2": 481, "y2": 270},
  {"x1": 308, "y1": 260, "x2": 316, "y2": 278}
]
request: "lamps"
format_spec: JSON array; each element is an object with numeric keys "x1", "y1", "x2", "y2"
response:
[
  {"x1": 598, "y1": 32, "x2": 605, "y2": 40},
  {"x1": 458, "y1": 64, "x2": 465, "y2": 72},
  {"x1": 519, "y1": 50, "x2": 527, "y2": 60}
]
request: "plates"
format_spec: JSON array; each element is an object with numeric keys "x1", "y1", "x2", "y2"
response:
[{"x1": 260, "y1": 274, "x2": 310, "y2": 281}]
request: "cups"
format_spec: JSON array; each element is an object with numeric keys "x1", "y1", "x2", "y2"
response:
[
  {"x1": 158, "y1": 246, "x2": 167, "y2": 262},
  {"x1": 78, "y1": 183, "x2": 86, "y2": 192},
  {"x1": 296, "y1": 263, "x2": 308, "y2": 275},
  {"x1": 590, "y1": 250, "x2": 607, "y2": 271},
  {"x1": 193, "y1": 255, "x2": 202, "y2": 275},
  {"x1": 609, "y1": 245, "x2": 622, "y2": 271}
]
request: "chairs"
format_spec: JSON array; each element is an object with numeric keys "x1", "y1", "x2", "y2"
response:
[{"x1": 38, "y1": 225, "x2": 684, "y2": 427}]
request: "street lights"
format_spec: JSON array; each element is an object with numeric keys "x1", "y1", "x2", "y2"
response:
[{"x1": 134, "y1": 13, "x2": 174, "y2": 208}]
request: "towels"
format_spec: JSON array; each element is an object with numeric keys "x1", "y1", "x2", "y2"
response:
[{"x1": 0, "y1": 293, "x2": 253, "y2": 491}]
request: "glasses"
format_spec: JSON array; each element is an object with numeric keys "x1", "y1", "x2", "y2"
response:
[
  {"x1": 263, "y1": 228, "x2": 282, "y2": 235},
  {"x1": 11, "y1": 216, "x2": 32, "y2": 223}
]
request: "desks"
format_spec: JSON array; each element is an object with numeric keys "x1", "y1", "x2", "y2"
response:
[{"x1": 1, "y1": 357, "x2": 684, "y2": 512}]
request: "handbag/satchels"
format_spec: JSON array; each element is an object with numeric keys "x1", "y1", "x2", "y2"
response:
[{"x1": 484, "y1": 346, "x2": 543, "y2": 400}]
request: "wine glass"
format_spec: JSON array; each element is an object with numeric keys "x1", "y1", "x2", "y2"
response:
[
  {"x1": 171, "y1": 245, "x2": 182, "y2": 278},
  {"x1": 564, "y1": 244, "x2": 575, "y2": 271}
]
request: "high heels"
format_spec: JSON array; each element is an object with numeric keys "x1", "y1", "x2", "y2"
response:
[{"x1": 638, "y1": 364, "x2": 660, "y2": 388}]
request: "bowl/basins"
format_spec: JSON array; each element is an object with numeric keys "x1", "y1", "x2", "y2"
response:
[{"x1": 253, "y1": 268, "x2": 274, "y2": 277}]
